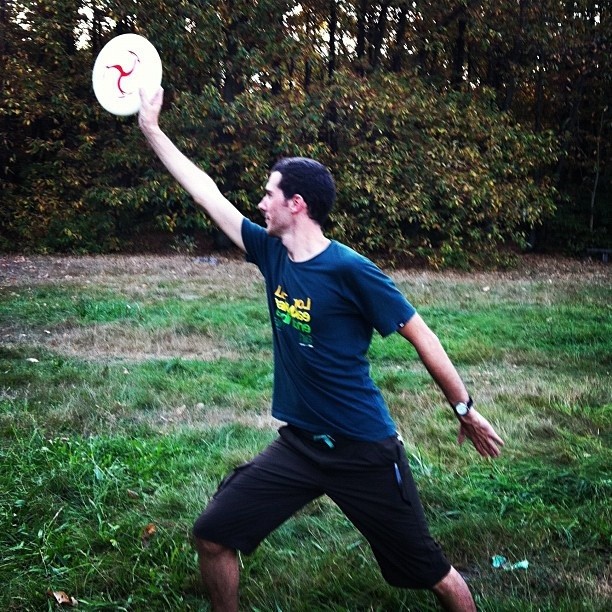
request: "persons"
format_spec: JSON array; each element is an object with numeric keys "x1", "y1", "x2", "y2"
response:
[{"x1": 138, "y1": 85, "x2": 504, "y2": 612}]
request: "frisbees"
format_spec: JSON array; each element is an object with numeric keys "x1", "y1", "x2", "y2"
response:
[{"x1": 92, "y1": 33, "x2": 163, "y2": 116}]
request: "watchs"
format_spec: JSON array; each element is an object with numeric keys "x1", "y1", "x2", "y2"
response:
[{"x1": 450, "y1": 397, "x2": 475, "y2": 415}]
